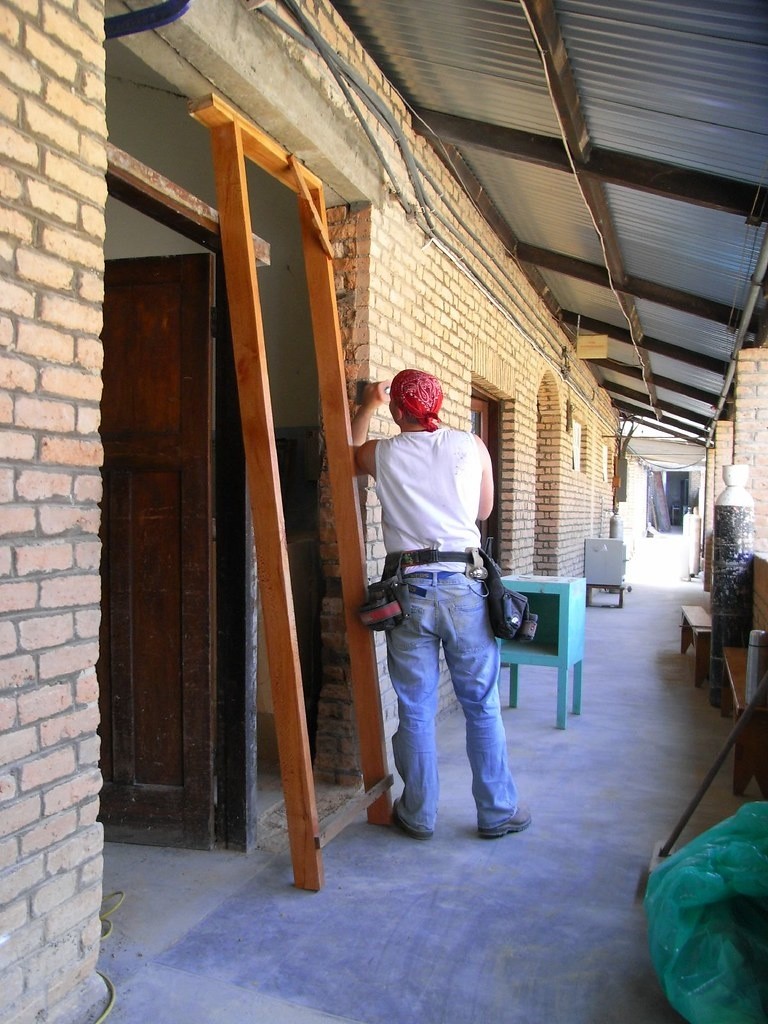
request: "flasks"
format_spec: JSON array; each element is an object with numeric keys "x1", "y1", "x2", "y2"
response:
[{"x1": 745, "y1": 630, "x2": 768, "y2": 706}]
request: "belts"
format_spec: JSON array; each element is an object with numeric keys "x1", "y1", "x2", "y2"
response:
[{"x1": 402, "y1": 571, "x2": 458, "y2": 598}]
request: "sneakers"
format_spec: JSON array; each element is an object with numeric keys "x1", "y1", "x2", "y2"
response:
[
  {"x1": 392, "y1": 797, "x2": 432, "y2": 841},
  {"x1": 477, "y1": 807, "x2": 531, "y2": 837}
]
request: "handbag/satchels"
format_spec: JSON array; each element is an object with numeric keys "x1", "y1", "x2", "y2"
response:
[{"x1": 481, "y1": 550, "x2": 528, "y2": 640}]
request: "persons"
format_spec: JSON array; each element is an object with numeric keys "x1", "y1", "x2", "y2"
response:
[{"x1": 351, "y1": 368, "x2": 532, "y2": 840}]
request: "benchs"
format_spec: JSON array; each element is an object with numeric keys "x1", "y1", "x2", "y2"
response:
[
  {"x1": 679, "y1": 606, "x2": 713, "y2": 689},
  {"x1": 718, "y1": 646, "x2": 768, "y2": 800}
]
